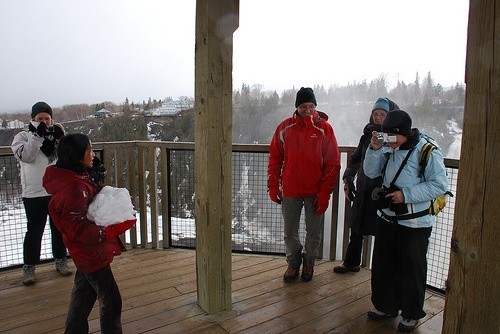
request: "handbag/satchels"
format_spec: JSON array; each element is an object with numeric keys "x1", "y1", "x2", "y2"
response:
[{"x1": 355, "y1": 197, "x2": 377, "y2": 230}]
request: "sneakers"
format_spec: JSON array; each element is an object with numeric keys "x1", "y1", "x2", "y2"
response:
[
  {"x1": 398, "y1": 317, "x2": 419, "y2": 332},
  {"x1": 55, "y1": 258, "x2": 73, "y2": 275},
  {"x1": 23, "y1": 265, "x2": 36, "y2": 283},
  {"x1": 369, "y1": 307, "x2": 398, "y2": 317}
]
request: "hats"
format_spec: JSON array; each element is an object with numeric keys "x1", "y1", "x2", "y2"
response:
[
  {"x1": 372, "y1": 97, "x2": 389, "y2": 113},
  {"x1": 31, "y1": 101, "x2": 53, "y2": 120},
  {"x1": 381, "y1": 110, "x2": 412, "y2": 136},
  {"x1": 295, "y1": 87, "x2": 317, "y2": 107}
]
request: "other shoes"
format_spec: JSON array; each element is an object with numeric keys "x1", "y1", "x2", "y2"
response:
[
  {"x1": 301, "y1": 264, "x2": 313, "y2": 279},
  {"x1": 334, "y1": 262, "x2": 360, "y2": 272},
  {"x1": 284, "y1": 267, "x2": 299, "y2": 280}
]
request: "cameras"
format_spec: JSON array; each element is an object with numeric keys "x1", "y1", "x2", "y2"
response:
[
  {"x1": 46, "y1": 125, "x2": 55, "y2": 132},
  {"x1": 377, "y1": 132, "x2": 397, "y2": 143}
]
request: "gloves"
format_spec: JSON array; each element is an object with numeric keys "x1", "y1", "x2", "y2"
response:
[
  {"x1": 106, "y1": 219, "x2": 136, "y2": 239},
  {"x1": 269, "y1": 187, "x2": 283, "y2": 205},
  {"x1": 36, "y1": 121, "x2": 48, "y2": 136},
  {"x1": 53, "y1": 124, "x2": 65, "y2": 141},
  {"x1": 343, "y1": 182, "x2": 356, "y2": 201},
  {"x1": 385, "y1": 183, "x2": 410, "y2": 215},
  {"x1": 311, "y1": 193, "x2": 330, "y2": 215}
]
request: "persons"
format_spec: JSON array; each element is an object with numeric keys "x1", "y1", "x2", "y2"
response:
[
  {"x1": 268, "y1": 87, "x2": 340, "y2": 282},
  {"x1": 334, "y1": 96, "x2": 400, "y2": 273},
  {"x1": 11, "y1": 102, "x2": 73, "y2": 284},
  {"x1": 363, "y1": 110, "x2": 448, "y2": 334},
  {"x1": 42, "y1": 134, "x2": 124, "y2": 334}
]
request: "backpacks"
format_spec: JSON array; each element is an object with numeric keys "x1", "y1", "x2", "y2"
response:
[{"x1": 419, "y1": 143, "x2": 454, "y2": 215}]
